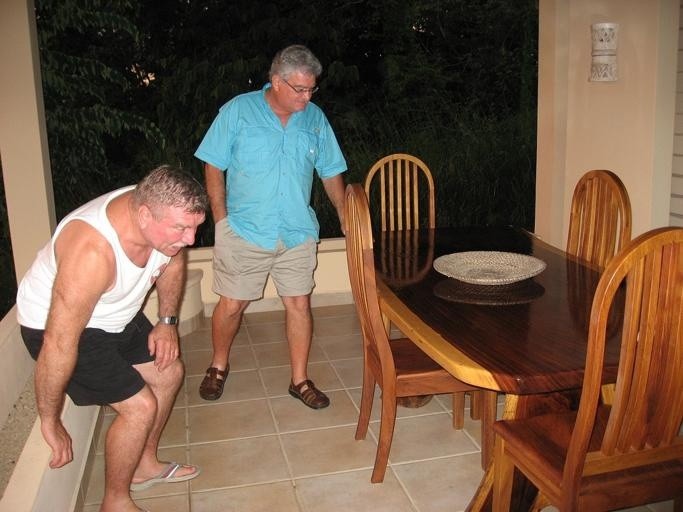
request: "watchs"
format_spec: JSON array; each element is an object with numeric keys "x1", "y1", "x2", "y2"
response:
[{"x1": 158, "y1": 314, "x2": 178, "y2": 325}]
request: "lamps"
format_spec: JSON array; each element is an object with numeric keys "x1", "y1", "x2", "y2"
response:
[{"x1": 587, "y1": 20, "x2": 621, "y2": 84}]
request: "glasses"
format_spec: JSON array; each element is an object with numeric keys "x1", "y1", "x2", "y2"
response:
[{"x1": 282, "y1": 81, "x2": 320, "y2": 96}]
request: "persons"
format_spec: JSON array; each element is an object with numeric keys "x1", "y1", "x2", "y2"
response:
[
  {"x1": 192, "y1": 44, "x2": 350, "y2": 411},
  {"x1": 15, "y1": 162, "x2": 212, "y2": 512}
]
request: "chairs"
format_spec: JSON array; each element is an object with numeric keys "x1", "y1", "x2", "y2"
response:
[
  {"x1": 367, "y1": 227, "x2": 435, "y2": 286},
  {"x1": 343, "y1": 183, "x2": 502, "y2": 484},
  {"x1": 363, "y1": 152, "x2": 436, "y2": 229},
  {"x1": 566, "y1": 168, "x2": 633, "y2": 270},
  {"x1": 492, "y1": 225, "x2": 683, "y2": 512}
]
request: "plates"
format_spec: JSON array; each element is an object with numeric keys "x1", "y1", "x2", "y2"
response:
[
  {"x1": 433, "y1": 249, "x2": 548, "y2": 288},
  {"x1": 431, "y1": 278, "x2": 547, "y2": 306}
]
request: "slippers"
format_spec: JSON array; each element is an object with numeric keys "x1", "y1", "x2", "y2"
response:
[{"x1": 128, "y1": 462, "x2": 203, "y2": 493}]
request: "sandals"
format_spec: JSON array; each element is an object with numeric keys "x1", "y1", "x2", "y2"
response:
[
  {"x1": 198, "y1": 359, "x2": 230, "y2": 401},
  {"x1": 289, "y1": 379, "x2": 332, "y2": 409}
]
